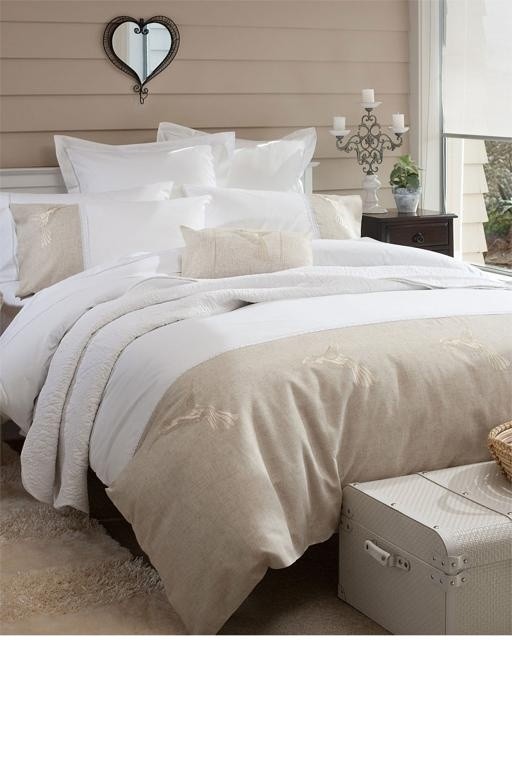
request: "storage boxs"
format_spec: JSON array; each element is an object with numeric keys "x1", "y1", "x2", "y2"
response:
[{"x1": 338, "y1": 454, "x2": 512, "y2": 633}]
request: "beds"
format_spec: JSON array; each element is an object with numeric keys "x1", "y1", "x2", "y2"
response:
[{"x1": 0, "y1": 160, "x2": 511, "y2": 633}]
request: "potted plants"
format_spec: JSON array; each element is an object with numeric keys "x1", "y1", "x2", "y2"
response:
[{"x1": 388, "y1": 154, "x2": 422, "y2": 214}]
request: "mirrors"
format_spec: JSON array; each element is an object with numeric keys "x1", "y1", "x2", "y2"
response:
[{"x1": 102, "y1": 13, "x2": 181, "y2": 104}]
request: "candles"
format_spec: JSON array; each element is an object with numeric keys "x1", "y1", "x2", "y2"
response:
[
  {"x1": 362, "y1": 88, "x2": 374, "y2": 104},
  {"x1": 392, "y1": 111, "x2": 405, "y2": 128},
  {"x1": 332, "y1": 115, "x2": 347, "y2": 131}
]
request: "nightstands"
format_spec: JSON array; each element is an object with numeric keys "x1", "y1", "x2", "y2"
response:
[{"x1": 360, "y1": 207, "x2": 456, "y2": 261}]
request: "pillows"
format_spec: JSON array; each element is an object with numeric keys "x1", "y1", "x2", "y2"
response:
[
  {"x1": 2, "y1": 181, "x2": 176, "y2": 282},
  {"x1": 182, "y1": 184, "x2": 362, "y2": 240},
  {"x1": 54, "y1": 131, "x2": 235, "y2": 195},
  {"x1": 9, "y1": 195, "x2": 205, "y2": 298},
  {"x1": 181, "y1": 225, "x2": 312, "y2": 280},
  {"x1": 156, "y1": 122, "x2": 317, "y2": 191}
]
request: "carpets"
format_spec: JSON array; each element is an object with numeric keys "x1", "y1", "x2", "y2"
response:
[{"x1": 0, "y1": 435, "x2": 192, "y2": 635}]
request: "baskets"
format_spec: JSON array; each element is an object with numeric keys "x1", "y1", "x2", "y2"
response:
[{"x1": 488, "y1": 422, "x2": 512, "y2": 482}]
